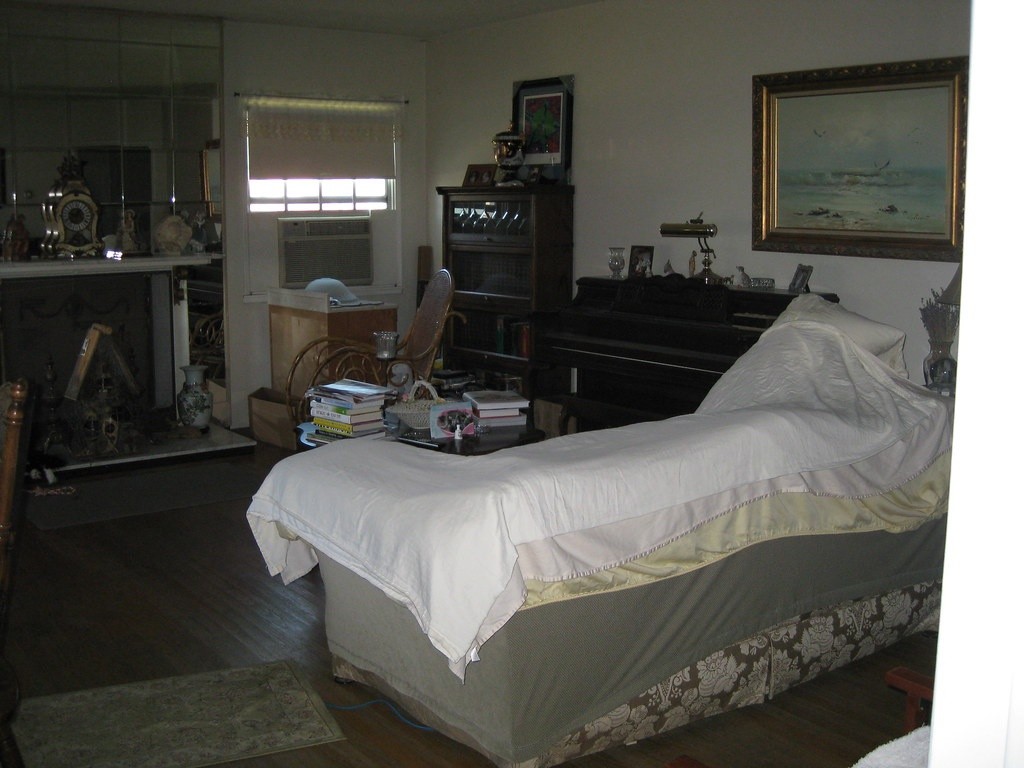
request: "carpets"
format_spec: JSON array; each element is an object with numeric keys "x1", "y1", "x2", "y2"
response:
[{"x1": 9, "y1": 658, "x2": 348, "y2": 767}]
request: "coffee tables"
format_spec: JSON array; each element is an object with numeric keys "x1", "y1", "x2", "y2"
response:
[{"x1": 293, "y1": 423, "x2": 546, "y2": 456}]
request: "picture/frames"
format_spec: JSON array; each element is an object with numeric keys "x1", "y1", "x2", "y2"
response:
[
  {"x1": 461, "y1": 163, "x2": 497, "y2": 187},
  {"x1": 751, "y1": 54, "x2": 969, "y2": 263},
  {"x1": 627, "y1": 245, "x2": 655, "y2": 280},
  {"x1": 510, "y1": 74, "x2": 574, "y2": 186},
  {"x1": 788, "y1": 263, "x2": 814, "y2": 294}
]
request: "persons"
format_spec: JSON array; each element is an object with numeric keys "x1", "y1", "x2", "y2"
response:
[
  {"x1": 117, "y1": 209, "x2": 136, "y2": 252},
  {"x1": 632, "y1": 257, "x2": 651, "y2": 274}
]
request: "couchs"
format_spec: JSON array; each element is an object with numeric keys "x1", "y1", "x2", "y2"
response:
[{"x1": 274, "y1": 389, "x2": 957, "y2": 768}]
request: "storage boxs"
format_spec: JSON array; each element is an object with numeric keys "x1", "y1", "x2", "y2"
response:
[{"x1": 249, "y1": 386, "x2": 306, "y2": 453}]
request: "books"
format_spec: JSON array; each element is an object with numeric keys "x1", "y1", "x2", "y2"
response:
[
  {"x1": 463, "y1": 390, "x2": 530, "y2": 427},
  {"x1": 496, "y1": 315, "x2": 531, "y2": 358},
  {"x1": 304, "y1": 378, "x2": 396, "y2": 444}
]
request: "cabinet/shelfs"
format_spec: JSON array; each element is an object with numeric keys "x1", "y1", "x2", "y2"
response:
[
  {"x1": 265, "y1": 288, "x2": 399, "y2": 397},
  {"x1": 1, "y1": 251, "x2": 260, "y2": 481},
  {"x1": 435, "y1": 186, "x2": 574, "y2": 378}
]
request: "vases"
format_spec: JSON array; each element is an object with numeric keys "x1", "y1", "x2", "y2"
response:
[
  {"x1": 922, "y1": 340, "x2": 957, "y2": 398},
  {"x1": 609, "y1": 247, "x2": 625, "y2": 278},
  {"x1": 176, "y1": 366, "x2": 214, "y2": 433}
]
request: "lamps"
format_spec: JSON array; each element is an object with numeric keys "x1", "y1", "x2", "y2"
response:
[{"x1": 658, "y1": 212, "x2": 722, "y2": 284}]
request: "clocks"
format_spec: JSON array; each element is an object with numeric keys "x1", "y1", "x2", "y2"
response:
[{"x1": 50, "y1": 150, "x2": 106, "y2": 259}]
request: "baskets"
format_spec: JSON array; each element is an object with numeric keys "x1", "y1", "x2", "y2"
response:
[{"x1": 385, "y1": 379, "x2": 456, "y2": 428}]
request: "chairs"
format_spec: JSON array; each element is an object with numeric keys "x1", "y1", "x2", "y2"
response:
[
  {"x1": 848, "y1": 664, "x2": 937, "y2": 767},
  {"x1": 283, "y1": 268, "x2": 454, "y2": 428},
  {"x1": 0, "y1": 377, "x2": 27, "y2": 768}
]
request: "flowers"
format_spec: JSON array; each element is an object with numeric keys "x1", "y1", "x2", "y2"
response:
[{"x1": 920, "y1": 286, "x2": 960, "y2": 393}]
